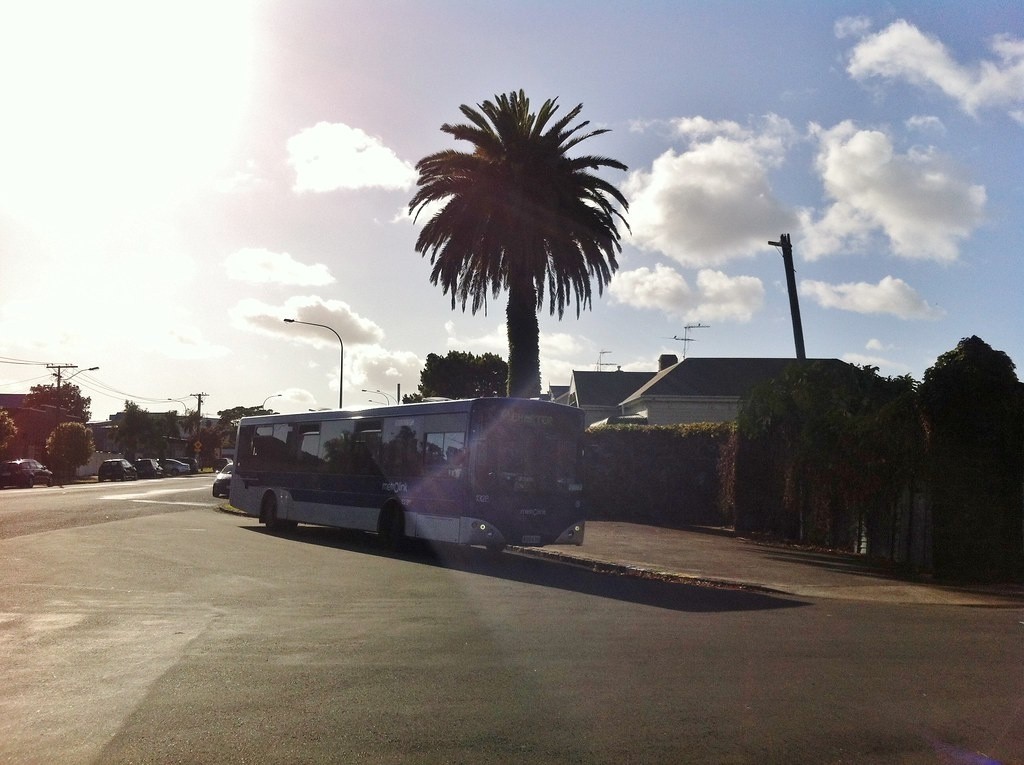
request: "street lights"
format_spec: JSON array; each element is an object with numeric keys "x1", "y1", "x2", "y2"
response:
[
  {"x1": 55, "y1": 367, "x2": 99, "y2": 489},
  {"x1": 168, "y1": 398, "x2": 187, "y2": 427},
  {"x1": 362, "y1": 388, "x2": 399, "y2": 406},
  {"x1": 262, "y1": 395, "x2": 283, "y2": 411},
  {"x1": 283, "y1": 319, "x2": 343, "y2": 411}
]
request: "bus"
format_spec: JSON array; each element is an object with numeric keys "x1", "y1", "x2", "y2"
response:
[
  {"x1": 212, "y1": 458, "x2": 233, "y2": 473},
  {"x1": 230, "y1": 394, "x2": 588, "y2": 553}
]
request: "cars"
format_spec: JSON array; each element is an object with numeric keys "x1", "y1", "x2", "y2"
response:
[
  {"x1": 133, "y1": 457, "x2": 201, "y2": 479},
  {"x1": 212, "y1": 463, "x2": 235, "y2": 496},
  {"x1": 0, "y1": 457, "x2": 54, "y2": 489},
  {"x1": 98, "y1": 458, "x2": 138, "y2": 481}
]
